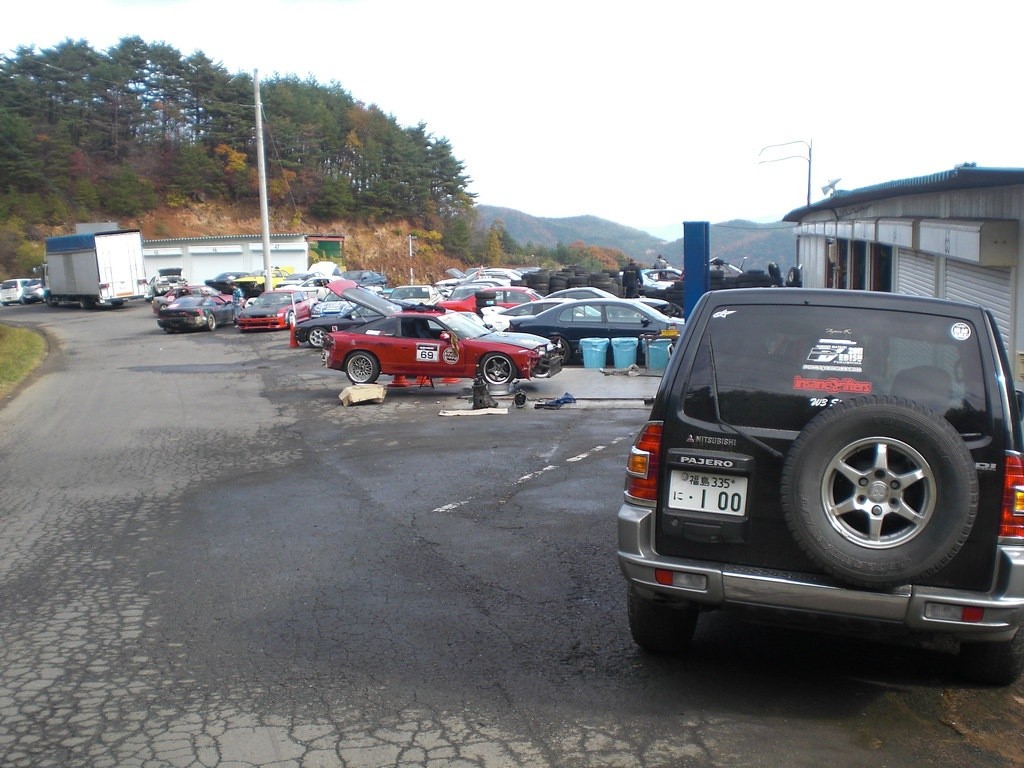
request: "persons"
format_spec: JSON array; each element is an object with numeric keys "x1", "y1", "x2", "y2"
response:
[
  {"x1": 654, "y1": 255, "x2": 669, "y2": 278},
  {"x1": 228, "y1": 283, "x2": 245, "y2": 329},
  {"x1": 621, "y1": 258, "x2": 644, "y2": 300}
]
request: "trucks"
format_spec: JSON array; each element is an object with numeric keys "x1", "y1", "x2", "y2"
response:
[{"x1": 43, "y1": 228, "x2": 146, "y2": 308}]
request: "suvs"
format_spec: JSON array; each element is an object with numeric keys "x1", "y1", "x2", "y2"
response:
[{"x1": 615, "y1": 285, "x2": 1023, "y2": 690}]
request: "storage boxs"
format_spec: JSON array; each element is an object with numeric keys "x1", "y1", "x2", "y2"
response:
[{"x1": 338, "y1": 384, "x2": 386, "y2": 406}]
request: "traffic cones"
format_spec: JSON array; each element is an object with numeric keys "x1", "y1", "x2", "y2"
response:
[
  {"x1": 411, "y1": 375, "x2": 431, "y2": 386},
  {"x1": 387, "y1": 372, "x2": 409, "y2": 387},
  {"x1": 288, "y1": 322, "x2": 299, "y2": 347},
  {"x1": 437, "y1": 377, "x2": 461, "y2": 384}
]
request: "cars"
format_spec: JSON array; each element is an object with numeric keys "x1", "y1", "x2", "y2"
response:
[
  {"x1": 279, "y1": 274, "x2": 343, "y2": 297},
  {"x1": 311, "y1": 287, "x2": 358, "y2": 318},
  {"x1": 232, "y1": 264, "x2": 294, "y2": 293},
  {"x1": 237, "y1": 289, "x2": 311, "y2": 331},
  {"x1": 276, "y1": 261, "x2": 337, "y2": 288},
  {"x1": 340, "y1": 268, "x2": 386, "y2": 289},
  {"x1": 434, "y1": 283, "x2": 542, "y2": 312},
  {"x1": 383, "y1": 265, "x2": 541, "y2": 304},
  {"x1": 151, "y1": 283, "x2": 246, "y2": 310},
  {"x1": 204, "y1": 271, "x2": 247, "y2": 291},
  {"x1": 541, "y1": 286, "x2": 669, "y2": 315},
  {"x1": 147, "y1": 275, "x2": 188, "y2": 301},
  {"x1": 294, "y1": 299, "x2": 406, "y2": 349},
  {"x1": 320, "y1": 279, "x2": 563, "y2": 387},
  {"x1": 509, "y1": 297, "x2": 686, "y2": 364},
  {"x1": 157, "y1": 293, "x2": 234, "y2": 332},
  {"x1": 23, "y1": 279, "x2": 50, "y2": 304},
  {"x1": 642, "y1": 268, "x2": 684, "y2": 289}
]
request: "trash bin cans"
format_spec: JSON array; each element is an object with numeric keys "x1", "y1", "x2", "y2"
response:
[
  {"x1": 579, "y1": 337, "x2": 610, "y2": 370},
  {"x1": 642, "y1": 337, "x2": 673, "y2": 369},
  {"x1": 611, "y1": 337, "x2": 639, "y2": 368}
]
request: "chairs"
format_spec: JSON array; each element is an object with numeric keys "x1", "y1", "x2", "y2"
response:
[
  {"x1": 738, "y1": 354, "x2": 807, "y2": 398},
  {"x1": 413, "y1": 320, "x2": 434, "y2": 339},
  {"x1": 890, "y1": 365, "x2": 952, "y2": 412},
  {"x1": 396, "y1": 322, "x2": 417, "y2": 338}
]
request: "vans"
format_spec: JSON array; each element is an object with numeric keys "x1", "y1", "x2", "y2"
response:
[{"x1": 0, "y1": 279, "x2": 32, "y2": 306}]
